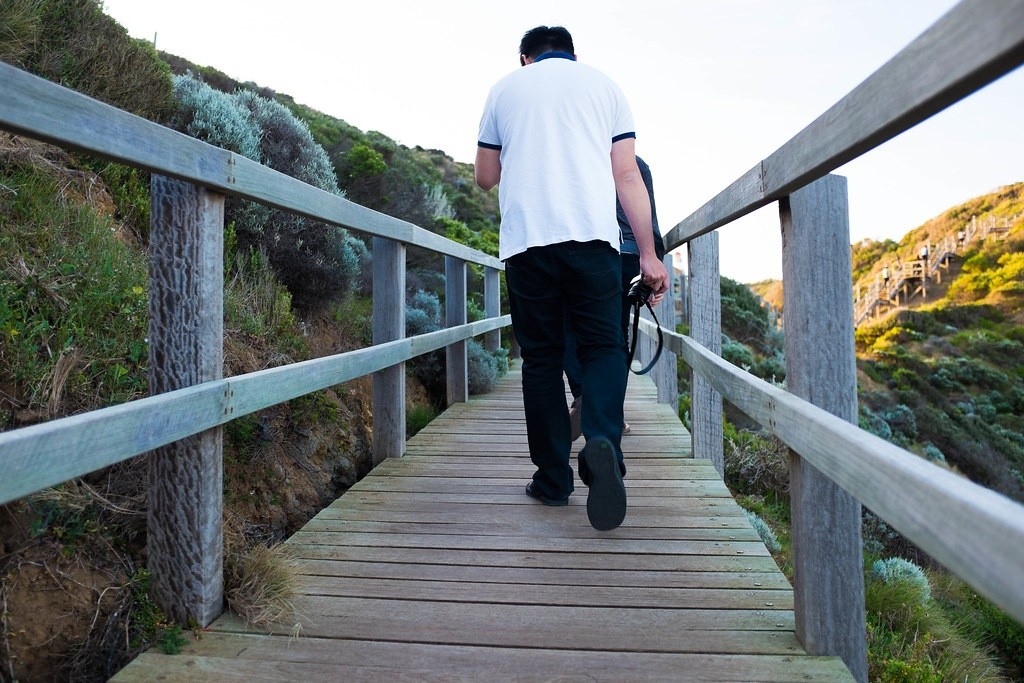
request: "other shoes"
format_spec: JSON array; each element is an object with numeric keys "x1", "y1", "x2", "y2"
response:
[
  {"x1": 569, "y1": 395, "x2": 583, "y2": 441},
  {"x1": 526, "y1": 480, "x2": 570, "y2": 507},
  {"x1": 584, "y1": 436, "x2": 626, "y2": 531}
]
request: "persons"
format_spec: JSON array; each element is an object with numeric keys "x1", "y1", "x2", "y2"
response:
[
  {"x1": 473, "y1": 25, "x2": 671, "y2": 532},
  {"x1": 565, "y1": 154, "x2": 666, "y2": 443}
]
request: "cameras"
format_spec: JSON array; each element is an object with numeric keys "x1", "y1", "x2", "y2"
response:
[{"x1": 626, "y1": 273, "x2": 654, "y2": 309}]
